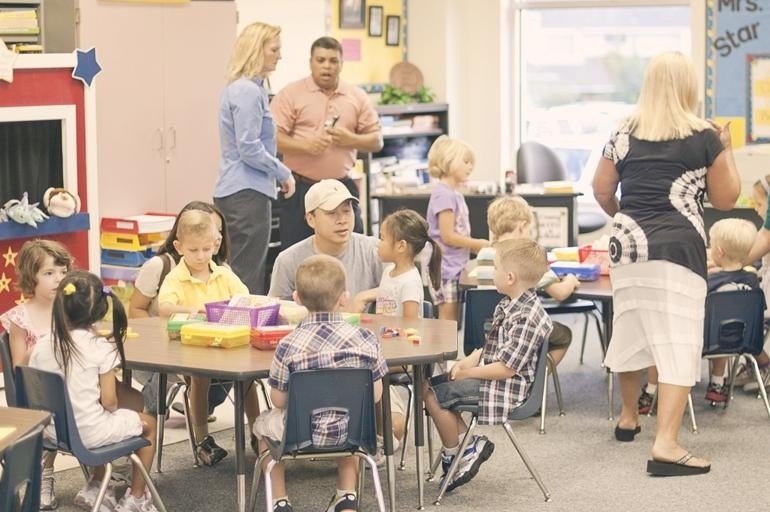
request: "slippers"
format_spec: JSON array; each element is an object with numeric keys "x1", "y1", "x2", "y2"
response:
[
  {"x1": 647, "y1": 453, "x2": 712, "y2": 474},
  {"x1": 614, "y1": 423, "x2": 642, "y2": 441},
  {"x1": 172, "y1": 402, "x2": 216, "y2": 423}
]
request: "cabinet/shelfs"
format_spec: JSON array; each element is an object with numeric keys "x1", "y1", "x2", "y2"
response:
[
  {"x1": 80, "y1": 1, "x2": 236, "y2": 218},
  {"x1": 357, "y1": 102, "x2": 450, "y2": 192}
]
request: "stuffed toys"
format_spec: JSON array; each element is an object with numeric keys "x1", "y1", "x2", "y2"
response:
[
  {"x1": 43, "y1": 187, "x2": 81, "y2": 218},
  {"x1": 0, "y1": 191, "x2": 48, "y2": 227}
]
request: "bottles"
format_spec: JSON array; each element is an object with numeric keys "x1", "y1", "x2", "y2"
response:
[{"x1": 505, "y1": 167, "x2": 513, "y2": 193}]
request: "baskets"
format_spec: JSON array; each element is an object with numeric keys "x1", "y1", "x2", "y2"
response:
[
  {"x1": 205, "y1": 299, "x2": 281, "y2": 326},
  {"x1": 578, "y1": 245, "x2": 609, "y2": 276}
]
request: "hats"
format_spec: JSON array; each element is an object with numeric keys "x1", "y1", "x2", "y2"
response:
[{"x1": 304, "y1": 178, "x2": 360, "y2": 213}]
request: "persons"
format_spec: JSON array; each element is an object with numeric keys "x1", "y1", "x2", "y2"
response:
[
  {"x1": 353, "y1": 210, "x2": 442, "y2": 453},
  {"x1": 422, "y1": 238, "x2": 551, "y2": 492},
  {"x1": 268, "y1": 37, "x2": 384, "y2": 251},
  {"x1": 266, "y1": 178, "x2": 392, "y2": 312},
  {"x1": 592, "y1": 52, "x2": 741, "y2": 476},
  {"x1": 428, "y1": 133, "x2": 490, "y2": 373},
  {"x1": 253, "y1": 255, "x2": 390, "y2": 511},
  {"x1": 639, "y1": 177, "x2": 769, "y2": 414},
  {"x1": 1, "y1": 238, "x2": 117, "y2": 511},
  {"x1": 478, "y1": 196, "x2": 580, "y2": 415},
  {"x1": 129, "y1": 203, "x2": 250, "y2": 468},
  {"x1": 28, "y1": 270, "x2": 158, "y2": 511},
  {"x1": 212, "y1": 23, "x2": 296, "y2": 296}
]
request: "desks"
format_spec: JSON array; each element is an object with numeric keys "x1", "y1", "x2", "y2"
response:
[
  {"x1": 368, "y1": 183, "x2": 585, "y2": 247},
  {"x1": 0, "y1": 407, "x2": 51, "y2": 461},
  {"x1": 460, "y1": 249, "x2": 762, "y2": 382},
  {"x1": 92, "y1": 311, "x2": 459, "y2": 512}
]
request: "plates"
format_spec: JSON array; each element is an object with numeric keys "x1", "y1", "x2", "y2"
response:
[{"x1": 390, "y1": 62, "x2": 423, "y2": 96}]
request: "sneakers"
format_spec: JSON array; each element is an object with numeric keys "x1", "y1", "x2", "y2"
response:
[
  {"x1": 273, "y1": 499, "x2": 293, "y2": 511},
  {"x1": 113, "y1": 488, "x2": 158, "y2": 511},
  {"x1": 39, "y1": 468, "x2": 57, "y2": 507},
  {"x1": 637, "y1": 364, "x2": 770, "y2": 414},
  {"x1": 365, "y1": 435, "x2": 399, "y2": 468},
  {"x1": 72, "y1": 475, "x2": 117, "y2": 512},
  {"x1": 195, "y1": 435, "x2": 229, "y2": 467},
  {"x1": 326, "y1": 489, "x2": 358, "y2": 511},
  {"x1": 439, "y1": 436, "x2": 494, "y2": 492}
]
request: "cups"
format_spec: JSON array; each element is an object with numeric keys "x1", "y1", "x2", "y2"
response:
[{"x1": 415, "y1": 167, "x2": 432, "y2": 190}]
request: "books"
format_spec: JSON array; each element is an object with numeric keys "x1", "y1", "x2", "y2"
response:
[
  {"x1": 357, "y1": 115, "x2": 443, "y2": 187},
  {"x1": 0, "y1": 9, "x2": 41, "y2": 52}
]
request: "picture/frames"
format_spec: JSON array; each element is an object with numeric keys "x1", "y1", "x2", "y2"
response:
[
  {"x1": 339, "y1": 0, "x2": 365, "y2": 29},
  {"x1": 386, "y1": 15, "x2": 400, "y2": 46},
  {"x1": 368, "y1": 5, "x2": 383, "y2": 37}
]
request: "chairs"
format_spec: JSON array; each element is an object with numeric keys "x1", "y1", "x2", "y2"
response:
[
  {"x1": 0, "y1": 425, "x2": 45, "y2": 512},
  {"x1": 426, "y1": 326, "x2": 553, "y2": 505},
  {"x1": 516, "y1": 142, "x2": 607, "y2": 233},
  {"x1": 0, "y1": 330, "x2": 16, "y2": 406},
  {"x1": 533, "y1": 294, "x2": 607, "y2": 367},
  {"x1": 369, "y1": 300, "x2": 434, "y2": 473},
  {"x1": 15, "y1": 366, "x2": 169, "y2": 512},
  {"x1": 247, "y1": 367, "x2": 386, "y2": 512},
  {"x1": 463, "y1": 288, "x2": 565, "y2": 436},
  {"x1": 647, "y1": 289, "x2": 770, "y2": 434},
  {"x1": 165, "y1": 372, "x2": 273, "y2": 468}
]
